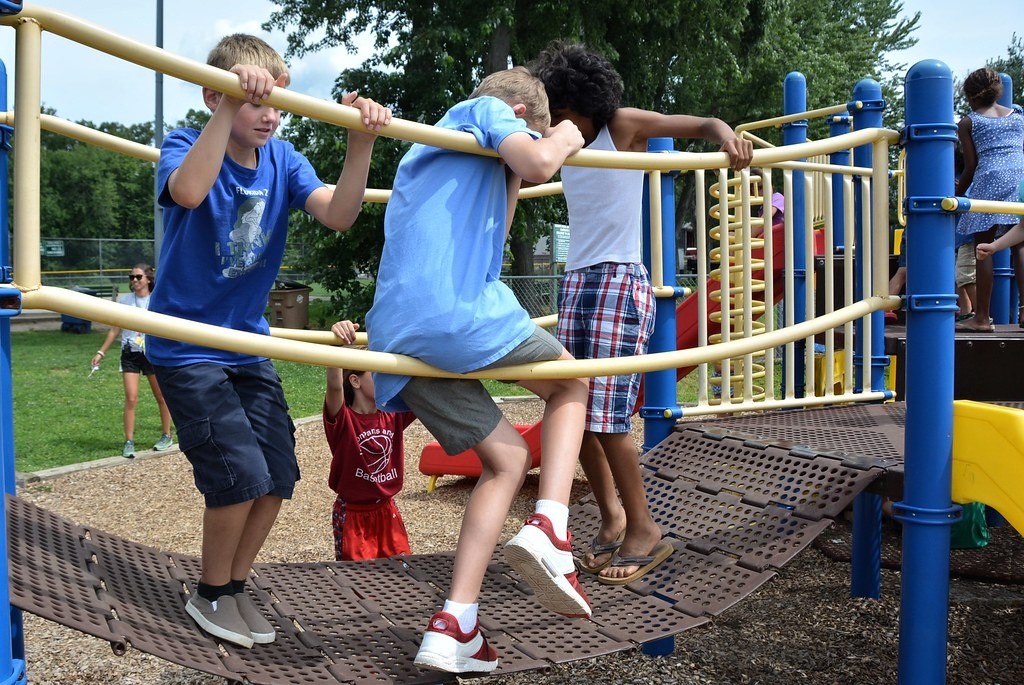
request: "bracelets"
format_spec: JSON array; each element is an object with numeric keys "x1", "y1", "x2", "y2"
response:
[{"x1": 96, "y1": 351, "x2": 105, "y2": 357}]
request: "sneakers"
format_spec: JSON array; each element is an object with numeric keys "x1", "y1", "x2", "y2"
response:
[
  {"x1": 122, "y1": 440, "x2": 135, "y2": 458},
  {"x1": 153, "y1": 434, "x2": 172, "y2": 451},
  {"x1": 184, "y1": 590, "x2": 255, "y2": 650},
  {"x1": 501, "y1": 513, "x2": 591, "y2": 617},
  {"x1": 413, "y1": 612, "x2": 499, "y2": 675},
  {"x1": 234, "y1": 592, "x2": 276, "y2": 644}
]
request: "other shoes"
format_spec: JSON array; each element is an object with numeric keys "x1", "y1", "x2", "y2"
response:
[
  {"x1": 955, "y1": 321, "x2": 994, "y2": 334},
  {"x1": 884, "y1": 311, "x2": 897, "y2": 322}
]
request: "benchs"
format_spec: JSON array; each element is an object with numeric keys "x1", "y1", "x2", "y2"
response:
[{"x1": 91, "y1": 283, "x2": 120, "y2": 301}]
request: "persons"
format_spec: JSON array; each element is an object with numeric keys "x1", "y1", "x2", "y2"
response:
[
  {"x1": 884, "y1": 70, "x2": 1024, "y2": 333},
  {"x1": 366, "y1": 68, "x2": 592, "y2": 675},
  {"x1": 323, "y1": 319, "x2": 418, "y2": 561},
  {"x1": 750, "y1": 167, "x2": 784, "y2": 239},
  {"x1": 91, "y1": 263, "x2": 173, "y2": 458},
  {"x1": 146, "y1": 35, "x2": 392, "y2": 648},
  {"x1": 523, "y1": 41, "x2": 754, "y2": 586}
]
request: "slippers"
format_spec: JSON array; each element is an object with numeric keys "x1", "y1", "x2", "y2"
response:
[
  {"x1": 596, "y1": 540, "x2": 673, "y2": 585},
  {"x1": 579, "y1": 527, "x2": 627, "y2": 573}
]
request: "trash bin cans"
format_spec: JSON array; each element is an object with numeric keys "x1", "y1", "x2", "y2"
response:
[
  {"x1": 61, "y1": 286, "x2": 97, "y2": 333},
  {"x1": 268, "y1": 280, "x2": 312, "y2": 329}
]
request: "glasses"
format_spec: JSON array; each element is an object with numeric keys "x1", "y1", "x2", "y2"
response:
[{"x1": 129, "y1": 274, "x2": 148, "y2": 281}]
